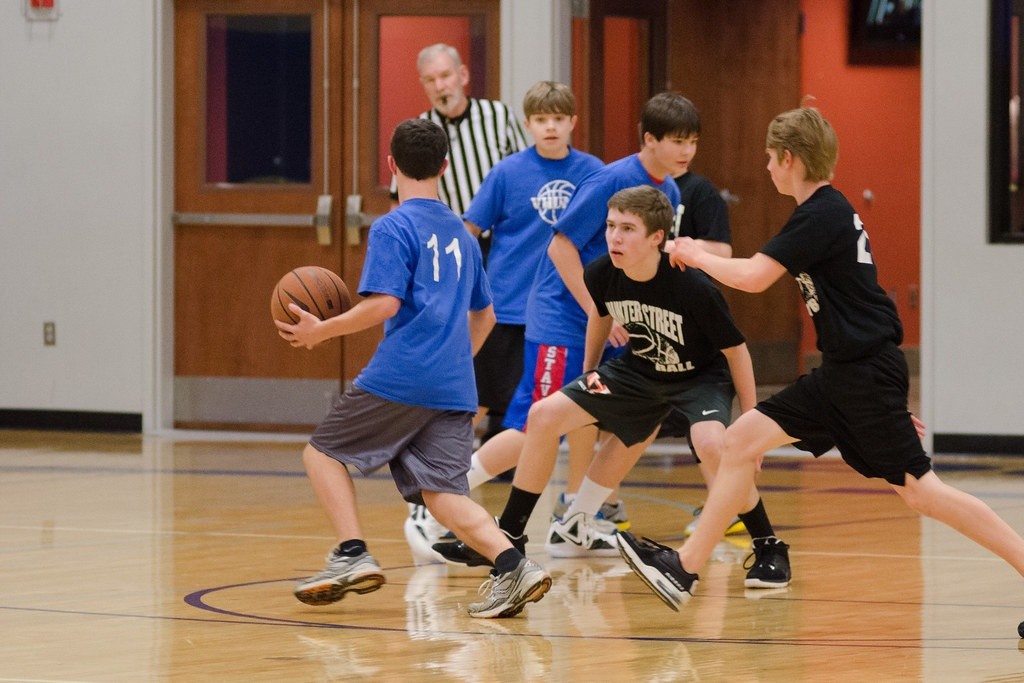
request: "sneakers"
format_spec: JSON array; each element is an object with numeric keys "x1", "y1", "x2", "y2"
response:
[
  {"x1": 743, "y1": 542, "x2": 793, "y2": 587},
  {"x1": 544, "y1": 511, "x2": 622, "y2": 558},
  {"x1": 404, "y1": 497, "x2": 448, "y2": 562},
  {"x1": 596, "y1": 499, "x2": 631, "y2": 530},
  {"x1": 467, "y1": 558, "x2": 552, "y2": 618},
  {"x1": 551, "y1": 492, "x2": 579, "y2": 519},
  {"x1": 430, "y1": 525, "x2": 529, "y2": 569},
  {"x1": 615, "y1": 531, "x2": 699, "y2": 612},
  {"x1": 293, "y1": 548, "x2": 385, "y2": 605},
  {"x1": 1018, "y1": 619, "x2": 1024, "y2": 637},
  {"x1": 684, "y1": 502, "x2": 748, "y2": 534}
]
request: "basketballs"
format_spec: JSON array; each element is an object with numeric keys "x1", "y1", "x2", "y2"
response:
[{"x1": 270, "y1": 265, "x2": 352, "y2": 345}]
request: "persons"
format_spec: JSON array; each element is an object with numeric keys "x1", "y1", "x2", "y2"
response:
[
  {"x1": 431, "y1": 184, "x2": 791, "y2": 589},
  {"x1": 616, "y1": 106, "x2": 1023, "y2": 650},
  {"x1": 273, "y1": 119, "x2": 551, "y2": 618},
  {"x1": 391, "y1": 44, "x2": 748, "y2": 563}
]
